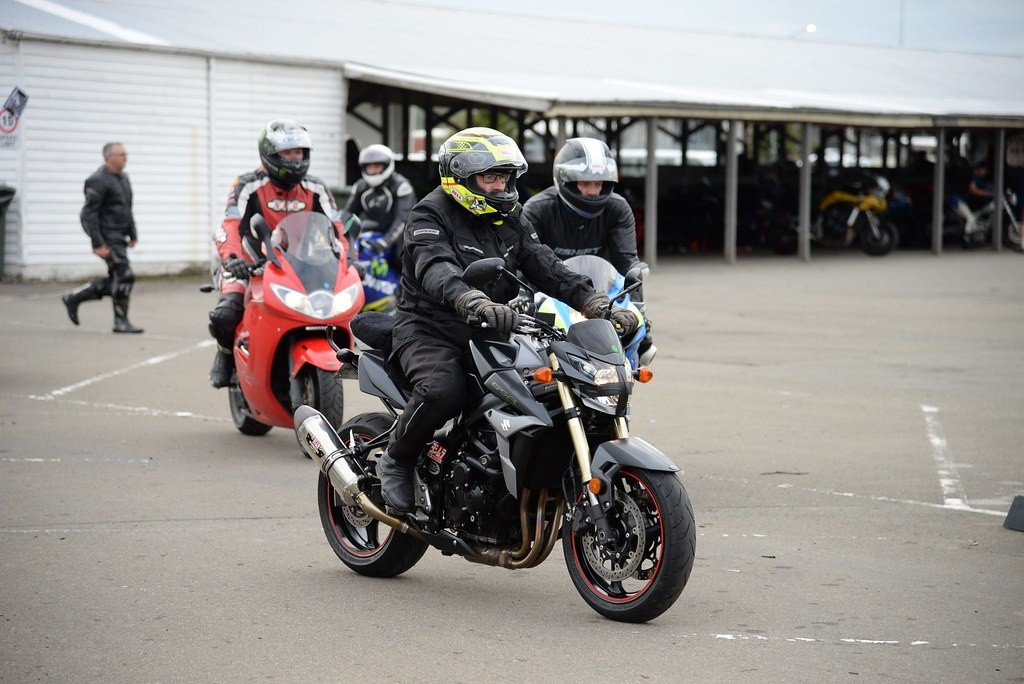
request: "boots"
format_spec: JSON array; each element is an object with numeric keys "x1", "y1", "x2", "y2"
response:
[
  {"x1": 62, "y1": 282, "x2": 100, "y2": 325},
  {"x1": 112, "y1": 299, "x2": 144, "y2": 332}
]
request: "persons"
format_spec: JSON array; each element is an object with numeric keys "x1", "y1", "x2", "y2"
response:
[
  {"x1": 737, "y1": 144, "x2": 828, "y2": 171},
  {"x1": 524, "y1": 137, "x2": 651, "y2": 333},
  {"x1": 62, "y1": 142, "x2": 145, "y2": 333},
  {"x1": 376, "y1": 126, "x2": 640, "y2": 511},
  {"x1": 209, "y1": 121, "x2": 364, "y2": 386},
  {"x1": 946, "y1": 162, "x2": 992, "y2": 249},
  {"x1": 910, "y1": 145, "x2": 966, "y2": 168},
  {"x1": 340, "y1": 143, "x2": 416, "y2": 278}
]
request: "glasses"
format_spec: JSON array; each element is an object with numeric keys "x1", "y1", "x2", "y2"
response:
[{"x1": 476, "y1": 173, "x2": 511, "y2": 183}]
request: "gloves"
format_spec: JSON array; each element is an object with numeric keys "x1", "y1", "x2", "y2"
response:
[
  {"x1": 454, "y1": 290, "x2": 519, "y2": 341},
  {"x1": 581, "y1": 292, "x2": 639, "y2": 336},
  {"x1": 366, "y1": 240, "x2": 386, "y2": 254},
  {"x1": 222, "y1": 253, "x2": 256, "y2": 279}
]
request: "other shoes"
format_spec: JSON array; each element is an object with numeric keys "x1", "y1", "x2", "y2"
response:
[{"x1": 209, "y1": 348, "x2": 234, "y2": 388}]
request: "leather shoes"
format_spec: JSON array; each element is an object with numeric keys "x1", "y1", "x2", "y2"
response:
[{"x1": 375, "y1": 447, "x2": 415, "y2": 512}]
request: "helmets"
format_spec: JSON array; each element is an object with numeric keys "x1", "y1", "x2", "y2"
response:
[
  {"x1": 438, "y1": 127, "x2": 525, "y2": 226},
  {"x1": 553, "y1": 137, "x2": 615, "y2": 220},
  {"x1": 258, "y1": 119, "x2": 312, "y2": 188},
  {"x1": 358, "y1": 144, "x2": 395, "y2": 187}
]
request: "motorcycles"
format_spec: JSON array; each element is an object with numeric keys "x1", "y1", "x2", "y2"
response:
[
  {"x1": 922, "y1": 178, "x2": 1024, "y2": 252},
  {"x1": 351, "y1": 233, "x2": 402, "y2": 322},
  {"x1": 820, "y1": 164, "x2": 900, "y2": 256},
  {"x1": 291, "y1": 254, "x2": 698, "y2": 625},
  {"x1": 198, "y1": 208, "x2": 367, "y2": 460},
  {"x1": 516, "y1": 255, "x2": 657, "y2": 426}
]
